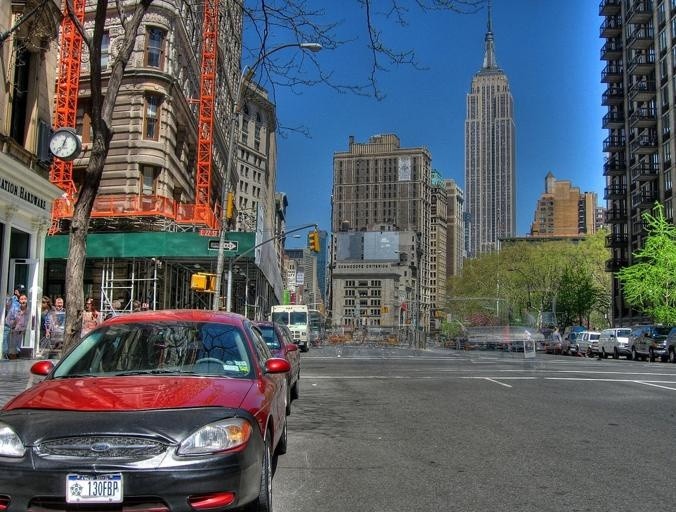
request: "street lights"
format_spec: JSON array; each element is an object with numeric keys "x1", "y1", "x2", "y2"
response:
[
  {"x1": 508, "y1": 269, "x2": 531, "y2": 311},
  {"x1": 211, "y1": 40, "x2": 324, "y2": 316},
  {"x1": 274, "y1": 235, "x2": 301, "y2": 245}
]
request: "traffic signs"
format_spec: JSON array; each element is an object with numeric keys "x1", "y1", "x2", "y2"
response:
[{"x1": 208, "y1": 239, "x2": 239, "y2": 252}]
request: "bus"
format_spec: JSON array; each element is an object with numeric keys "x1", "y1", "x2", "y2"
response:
[{"x1": 308, "y1": 309, "x2": 326, "y2": 343}]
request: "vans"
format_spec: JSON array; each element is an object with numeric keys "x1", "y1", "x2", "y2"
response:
[
  {"x1": 598, "y1": 328, "x2": 632, "y2": 359},
  {"x1": 269, "y1": 304, "x2": 310, "y2": 352},
  {"x1": 576, "y1": 331, "x2": 600, "y2": 357}
]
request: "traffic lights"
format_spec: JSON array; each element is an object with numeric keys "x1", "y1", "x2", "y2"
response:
[
  {"x1": 526, "y1": 302, "x2": 531, "y2": 310},
  {"x1": 306, "y1": 228, "x2": 320, "y2": 253}
]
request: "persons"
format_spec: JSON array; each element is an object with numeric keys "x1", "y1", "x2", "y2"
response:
[{"x1": 0, "y1": 278, "x2": 147, "y2": 363}]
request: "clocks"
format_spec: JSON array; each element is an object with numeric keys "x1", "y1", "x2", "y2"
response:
[{"x1": 48, "y1": 127, "x2": 83, "y2": 161}]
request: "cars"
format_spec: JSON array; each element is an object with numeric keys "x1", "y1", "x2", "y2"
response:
[
  {"x1": 0, "y1": 308, "x2": 293, "y2": 511},
  {"x1": 255, "y1": 322, "x2": 303, "y2": 415},
  {"x1": 465, "y1": 325, "x2": 588, "y2": 354},
  {"x1": 666, "y1": 327, "x2": 676, "y2": 363}
]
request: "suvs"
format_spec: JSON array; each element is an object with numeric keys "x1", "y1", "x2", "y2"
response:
[{"x1": 627, "y1": 323, "x2": 671, "y2": 361}]
